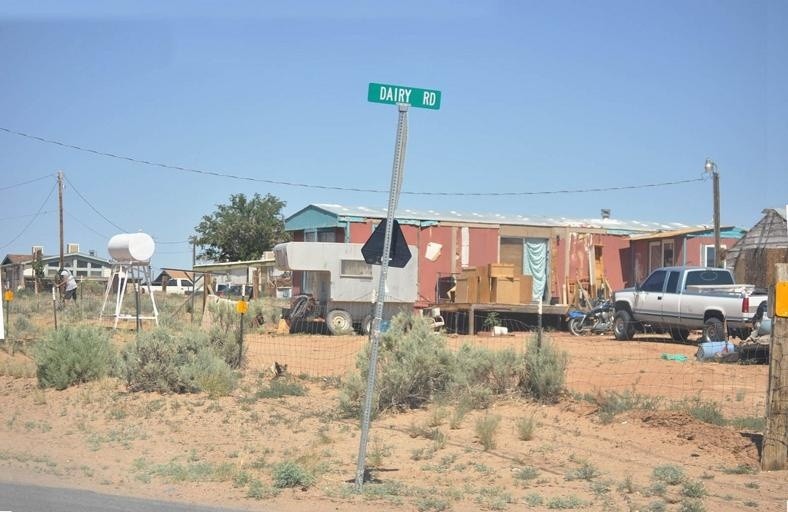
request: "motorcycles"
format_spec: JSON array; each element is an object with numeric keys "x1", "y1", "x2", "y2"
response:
[{"x1": 565, "y1": 300, "x2": 615, "y2": 335}]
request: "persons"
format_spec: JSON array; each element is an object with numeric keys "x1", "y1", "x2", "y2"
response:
[{"x1": 56, "y1": 267, "x2": 78, "y2": 306}]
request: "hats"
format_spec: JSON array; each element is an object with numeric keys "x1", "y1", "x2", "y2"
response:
[{"x1": 61, "y1": 271, "x2": 68, "y2": 276}]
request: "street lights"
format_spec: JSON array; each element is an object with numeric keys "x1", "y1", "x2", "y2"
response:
[{"x1": 705, "y1": 160, "x2": 721, "y2": 267}]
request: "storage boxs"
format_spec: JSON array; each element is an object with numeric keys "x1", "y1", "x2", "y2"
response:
[{"x1": 454, "y1": 262, "x2": 532, "y2": 305}]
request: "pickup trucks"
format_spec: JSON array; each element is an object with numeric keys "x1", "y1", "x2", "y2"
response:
[
  {"x1": 612, "y1": 266, "x2": 769, "y2": 342},
  {"x1": 140, "y1": 278, "x2": 204, "y2": 294}
]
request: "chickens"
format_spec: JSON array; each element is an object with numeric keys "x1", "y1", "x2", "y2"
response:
[{"x1": 275, "y1": 362, "x2": 288, "y2": 374}]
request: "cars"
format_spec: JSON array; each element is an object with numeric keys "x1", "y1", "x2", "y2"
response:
[{"x1": 216, "y1": 285, "x2": 253, "y2": 300}]
